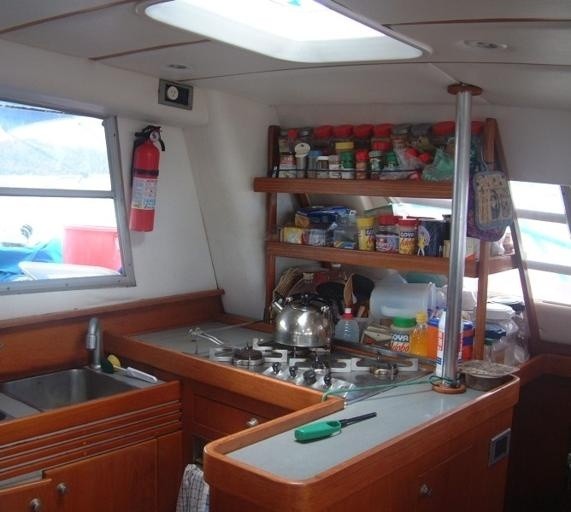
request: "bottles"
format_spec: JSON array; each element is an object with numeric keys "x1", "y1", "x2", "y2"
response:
[
  {"x1": 298, "y1": 272, "x2": 317, "y2": 294},
  {"x1": 327, "y1": 263, "x2": 346, "y2": 281},
  {"x1": 503, "y1": 232, "x2": 514, "y2": 255},
  {"x1": 389, "y1": 295, "x2": 528, "y2": 379},
  {"x1": 335, "y1": 307, "x2": 360, "y2": 342},
  {"x1": 272, "y1": 121, "x2": 484, "y2": 179},
  {"x1": 356, "y1": 214, "x2": 453, "y2": 258}
]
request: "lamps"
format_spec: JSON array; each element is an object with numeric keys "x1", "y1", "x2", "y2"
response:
[{"x1": 134, "y1": 0, "x2": 434, "y2": 65}]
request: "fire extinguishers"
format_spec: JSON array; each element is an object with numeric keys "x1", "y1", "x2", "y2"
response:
[{"x1": 128, "y1": 124, "x2": 165, "y2": 231}]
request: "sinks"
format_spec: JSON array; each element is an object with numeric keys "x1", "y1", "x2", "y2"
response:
[{"x1": 0, "y1": 368, "x2": 143, "y2": 413}]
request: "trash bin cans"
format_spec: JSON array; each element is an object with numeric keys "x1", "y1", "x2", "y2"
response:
[{"x1": 63, "y1": 225, "x2": 119, "y2": 271}]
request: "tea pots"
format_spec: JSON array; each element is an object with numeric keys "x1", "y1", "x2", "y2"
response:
[{"x1": 269, "y1": 292, "x2": 336, "y2": 346}]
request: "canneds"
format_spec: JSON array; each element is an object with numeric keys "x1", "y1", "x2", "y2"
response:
[{"x1": 399, "y1": 219, "x2": 418, "y2": 255}]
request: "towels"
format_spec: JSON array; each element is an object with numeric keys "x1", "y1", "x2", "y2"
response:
[{"x1": 175, "y1": 463, "x2": 210, "y2": 511}]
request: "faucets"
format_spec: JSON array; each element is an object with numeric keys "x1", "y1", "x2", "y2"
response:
[{"x1": 85, "y1": 318, "x2": 103, "y2": 370}]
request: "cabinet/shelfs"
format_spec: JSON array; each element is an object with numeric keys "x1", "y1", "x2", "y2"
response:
[
  {"x1": 252, "y1": 118, "x2": 570, "y2": 385},
  {"x1": 0, "y1": 379, "x2": 183, "y2": 512}
]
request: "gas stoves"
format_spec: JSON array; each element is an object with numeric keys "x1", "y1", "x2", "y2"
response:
[{"x1": 197, "y1": 337, "x2": 434, "y2": 402}]
